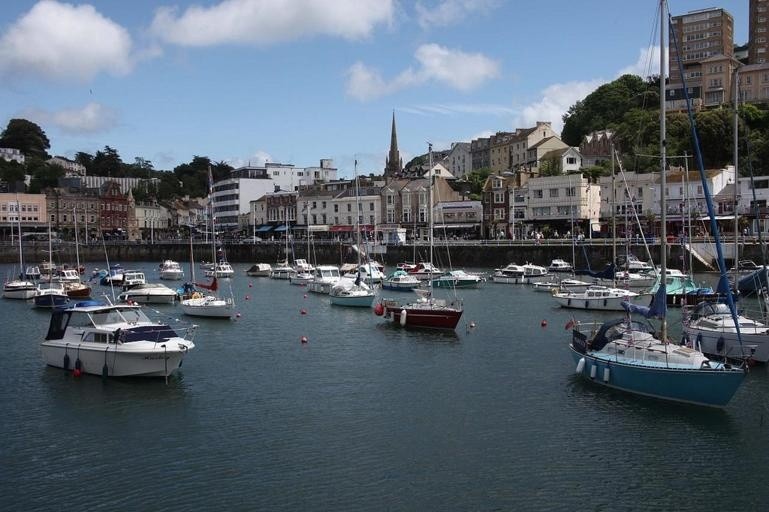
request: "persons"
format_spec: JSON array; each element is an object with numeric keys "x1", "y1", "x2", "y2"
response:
[
  {"x1": 667, "y1": 232, "x2": 674, "y2": 243},
  {"x1": 500, "y1": 229, "x2": 505, "y2": 240},
  {"x1": 577, "y1": 231, "x2": 583, "y2": 241},
  {"x1": 531, "y1": 229, "x2": 545, "y2": 240},
  {"x1": 581, "y1": 231, "x2": 586, "y2": 239},
  {"x1": 741, "y1": 225, "x2": 748, "y2": 241},
  {"x1": 684, "y1": 230, "x2": 688, "y2": 242},
  {"x1": 553, "y1": 229, "x2": 559, "y2": 239},
  {"x1": 678, "y1": 231, "x2": 684, "y2": 245}
]
